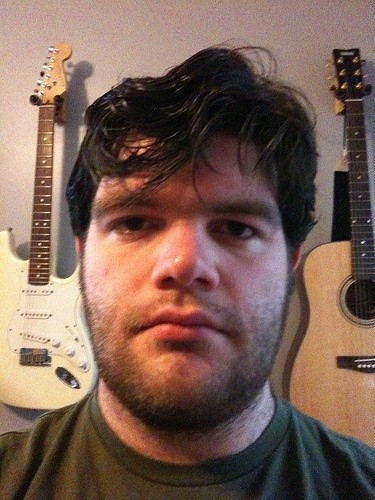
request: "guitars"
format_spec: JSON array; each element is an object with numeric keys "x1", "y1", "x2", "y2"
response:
[
  {"x1": 286, "y1": 47, "x2": 375, "y2": 450},
  {"x1": 0, "y1": 40, "x2": 99, "y2": 411}
]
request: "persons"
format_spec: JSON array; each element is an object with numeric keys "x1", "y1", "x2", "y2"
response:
[{"x1": 0, "y1": 42, "x2": 375, "y2": 499}]
